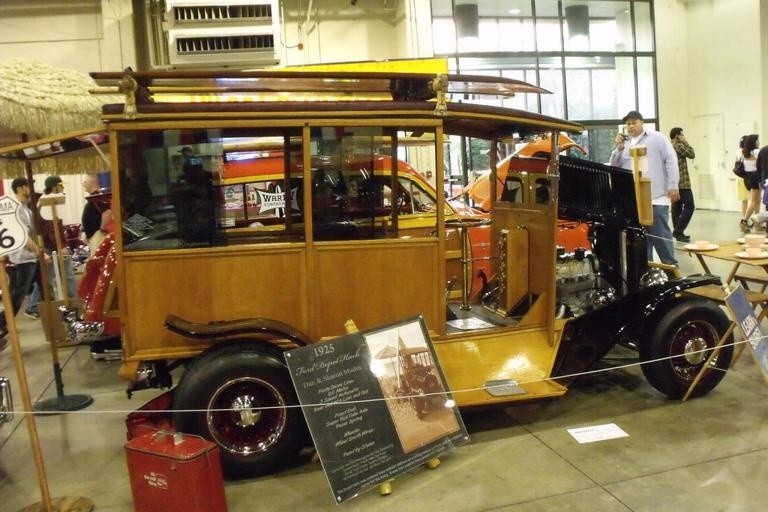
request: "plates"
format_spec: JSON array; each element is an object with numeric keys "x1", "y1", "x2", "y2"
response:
[
  {"x1": 734, "y1": 238, "x2": 768, "y2": 260},
  {"x1": 684, "y1": 244, "x2": 719, "y2": 250}
]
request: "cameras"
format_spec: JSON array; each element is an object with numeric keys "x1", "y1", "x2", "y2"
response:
[{"x1": 623, "y1": 135, "x2": 629, "y2": 140}]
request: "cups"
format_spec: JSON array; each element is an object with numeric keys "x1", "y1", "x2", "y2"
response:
[
  {"x1": 744, "y1": 234, "x2": 765, "y2": 248},
  {"x1": 696, "y1": 241, "x2": 709, "y2": 249}
]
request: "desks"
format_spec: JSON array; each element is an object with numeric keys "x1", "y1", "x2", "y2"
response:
[{"x1": 673, "y1": 239, "x2": 768, "y2": 403}]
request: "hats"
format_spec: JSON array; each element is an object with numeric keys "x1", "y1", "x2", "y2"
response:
[
  {"x1": 623, "y1": 111, "x2": 642, "y2": 120},
  {"x1": 44, "y1": 176, "x2": 62, "y2": 194},
  {"x1": 12, "y1": 177, "x2": 35, "y2": 189}
]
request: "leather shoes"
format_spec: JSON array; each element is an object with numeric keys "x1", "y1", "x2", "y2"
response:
[
  {"x1": 677, "y1": 237, "x2": 689, "y2": 242},
  {"x1": 673, "y1": 230, "x2": 690, "y2": 239}
]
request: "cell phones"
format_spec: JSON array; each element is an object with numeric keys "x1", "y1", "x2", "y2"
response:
[{"x1": 678, "y1": 135, "x2": 681, "y2": 139}]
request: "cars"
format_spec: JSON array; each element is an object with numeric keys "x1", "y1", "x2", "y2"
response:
[{"x1": 58, "y1": 133, "x2": 599, "y2": 363}]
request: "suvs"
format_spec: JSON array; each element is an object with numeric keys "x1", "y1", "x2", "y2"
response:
[{"x1": 81, "y1": 64, "x2": 734, "y2": 480}]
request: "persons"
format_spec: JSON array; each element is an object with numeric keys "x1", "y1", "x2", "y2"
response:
[
  {"x1": 740, "y1": 135, "x2": 762, "y2": 235},
  {"x1": 79, "y1": 176, "x2": 115, "y2": 255},
  {"x1": 622, "y1": 110, "x2": 682, "y2": 281},
  {"x1": 665, "y1": 125, "x2": 695, "y2": 245},
  {"x1": 734, "y1": 136, "x2": 750, "y2": 235},
  {"x1": 170, "y1": 146, "x2": 228, "y2": 249},
  {"x1": 0, "y1": 177, "x2": 36, "y2": 337},
  {"x1": 607, "y1": 134, "x2": 626, "y2": 169},
  {"x1": 755, "y1": 142, "x2": 768, "y2": 236},
  {"x1": 24, "y1": 172, "x2": 77, "y2": 321}
]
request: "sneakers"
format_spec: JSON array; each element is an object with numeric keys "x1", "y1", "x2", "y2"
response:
[
  {"x1": 755, "y1": 226, "x2": 767, "y2": 234},
  {"x1": 740, "y1": 220, "x2": 750, "y2": 233},
  {"x1": 24, "y1": 309, "x2": 40, "y2": 319}
]
